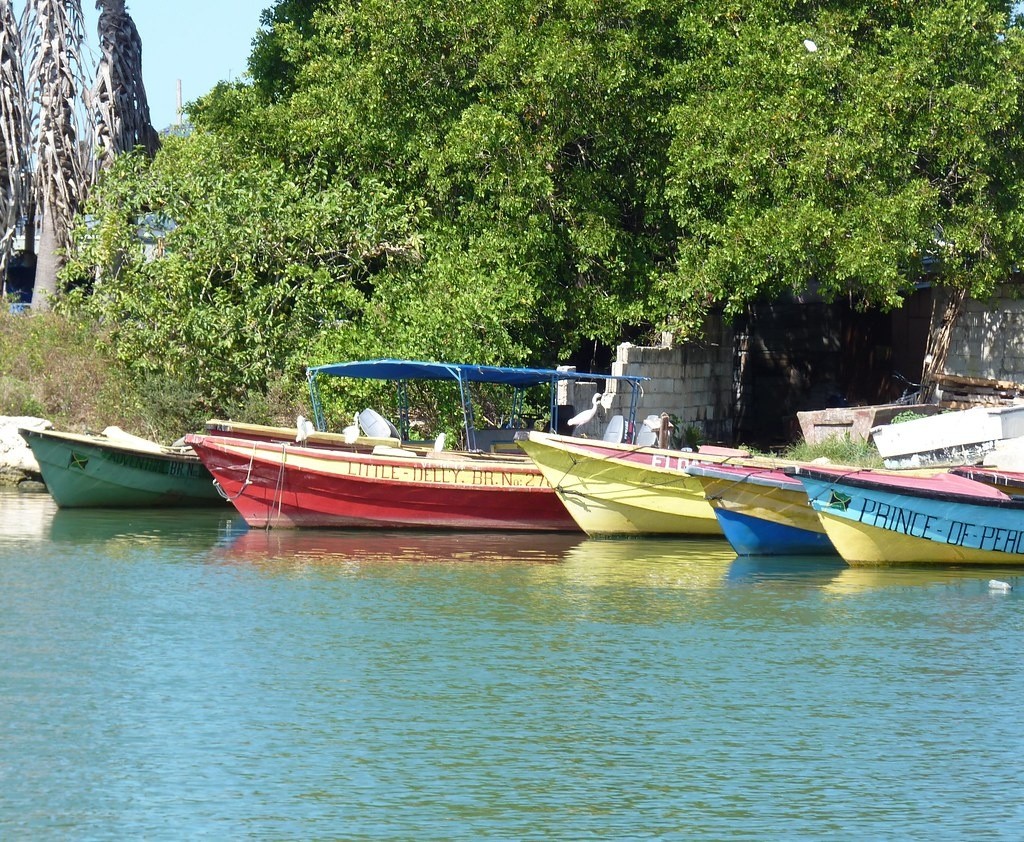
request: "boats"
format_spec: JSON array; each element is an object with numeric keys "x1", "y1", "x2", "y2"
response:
[
  {"x1": 685, "y1": 464, "x2": 837, "y2": 555},
  {"x1": 206, "y1": 358, "x2": 652, "y2": 463},
  {"x1": 183, "y1": 431, "x2": 581, "y2": 531},
  {"x1": 16, "y1": 423, "x2": 230, "y2": 510},
  {"x1": 512, "y1": 428, "x2": 797, "y2": 541},
  {"x1": 780, "y1": 466, "x2": 1024, "y2": 567}
]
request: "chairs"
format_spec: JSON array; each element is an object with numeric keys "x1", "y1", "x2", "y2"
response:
[
  {"x1": 603, "y1": 415, "x2": 625, "y2": 443},
  {"x1": 635, "y1": 414, "x2": 660, "y2": 446}
]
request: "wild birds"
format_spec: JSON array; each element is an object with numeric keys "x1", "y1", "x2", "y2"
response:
[
  {"x1": 294, "y1": 414, "x2": 317, "y2": 449},
  {"x1": 567, "y1": 392, "x2": 605, "y2": 439},
  {"x1": 341, "y1": 410, "x2": 362, "y2": 454},
  {"x1": 433, "y1": 432, "x2": 449, "y2": 455}
]
